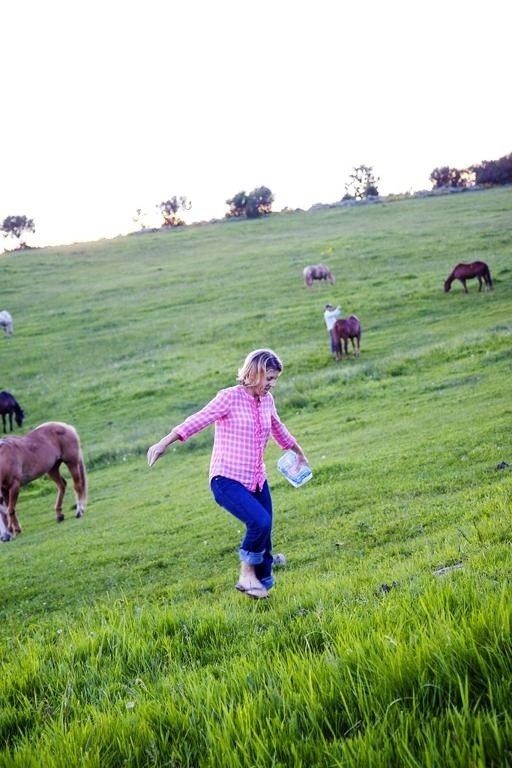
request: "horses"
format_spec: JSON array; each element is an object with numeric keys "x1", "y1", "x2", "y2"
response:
[
  {"x1": 0, "y1": 310, "x2": 13, "y2": 335},
  {"x1": 303, "y1": 263, "x2": 335, "y2": 290},
  {"x1": 0, "y1": 421, "x2": 88, "y2": 543},
  {"x1": 0, "y1": 391, "x2": 25, "y2": 432},
  {"x1": 332, "y1": 314, "x2": 361, "y2": 361},
  {"x1": 442, "y1": 260, "x2": 493, "y2": 293}
]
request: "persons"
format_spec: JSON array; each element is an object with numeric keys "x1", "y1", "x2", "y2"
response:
[
  {"x1": 144, "y1": 349, "x2": 310, "y2": 600},
  {"x1": 323, "y1": 302, "x2": 345, "y2": 364}
]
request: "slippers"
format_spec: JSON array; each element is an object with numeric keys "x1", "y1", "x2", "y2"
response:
[{"x1": 235, "y1": 583, "x2": 267, "y2": 598}]
